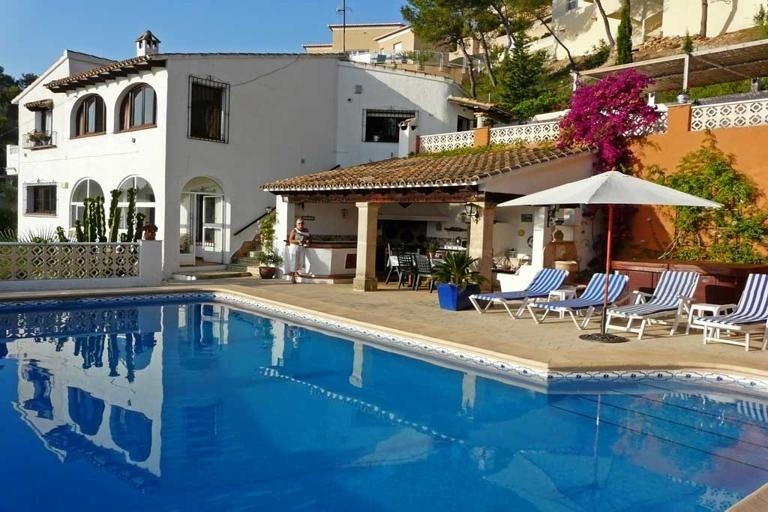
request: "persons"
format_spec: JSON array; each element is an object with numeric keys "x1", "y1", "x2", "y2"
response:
[
  {"x1": 285, "y1": 217, "x2": 310, "y2": 284},
  {"x1": 285, "y1": 323, "x2": 311, "y2": 350}
]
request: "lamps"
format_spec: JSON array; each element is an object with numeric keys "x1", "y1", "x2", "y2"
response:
[{"x1": 463, "y1": 202, "x2": 482, "y2": 225}]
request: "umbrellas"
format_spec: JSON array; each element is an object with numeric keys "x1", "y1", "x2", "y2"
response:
[
  {"x1": 481, "y1": 392, "x2": 709, "y2": 509},
  {"x1": 496, "y1": 163, "x2": 726, "y2": 333}
]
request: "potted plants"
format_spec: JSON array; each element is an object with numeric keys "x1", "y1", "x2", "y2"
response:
[
  {"x1": 252, "y1": 251, "x2": 283, "y2": 279},
  {"x1": 424, "y1": 250, "x2": 489, "y2": 312}
]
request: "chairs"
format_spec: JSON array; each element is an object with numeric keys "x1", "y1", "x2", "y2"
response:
[{"x1": 384, "y1": 242, "x2": 445, "y2": 293}]
